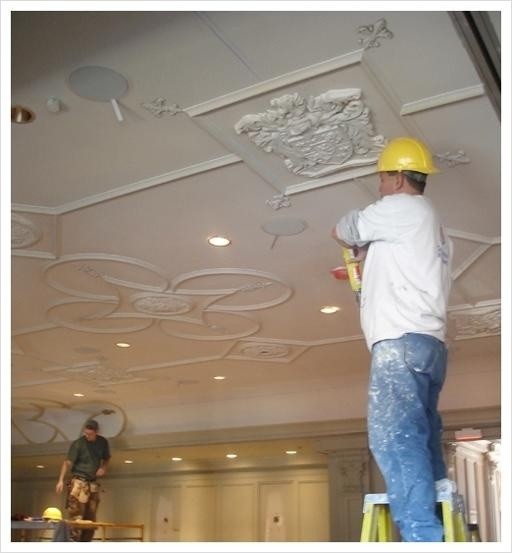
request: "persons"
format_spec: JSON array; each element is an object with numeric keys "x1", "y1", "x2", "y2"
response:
[
  {"x1": 54, "y1": 418, "x2": 113, "y2": 542},
  {"x1": 328, "y1": 136, "x2": 454, "y2": 543}
]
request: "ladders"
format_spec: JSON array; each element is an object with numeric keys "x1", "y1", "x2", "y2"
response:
[{"x1": 359, "y1": 479, "x2": 470, "y2": 542}]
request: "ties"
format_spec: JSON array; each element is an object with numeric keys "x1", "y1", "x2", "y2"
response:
[{"x1": 74, "y1": 475, "x2": 98, "y2": 482}]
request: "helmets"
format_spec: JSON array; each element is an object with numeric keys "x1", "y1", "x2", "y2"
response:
[
  {"x1": 42, "y1": 507, "x2": 63, "y2": 521},
  {"x1": 378, "y1": 137, "x2": 441, "y2": 176}
]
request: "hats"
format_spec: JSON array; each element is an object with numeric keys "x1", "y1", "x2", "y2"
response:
[{"x1": 84, "y1": 420, "x2": 100, "y2": 430}]
